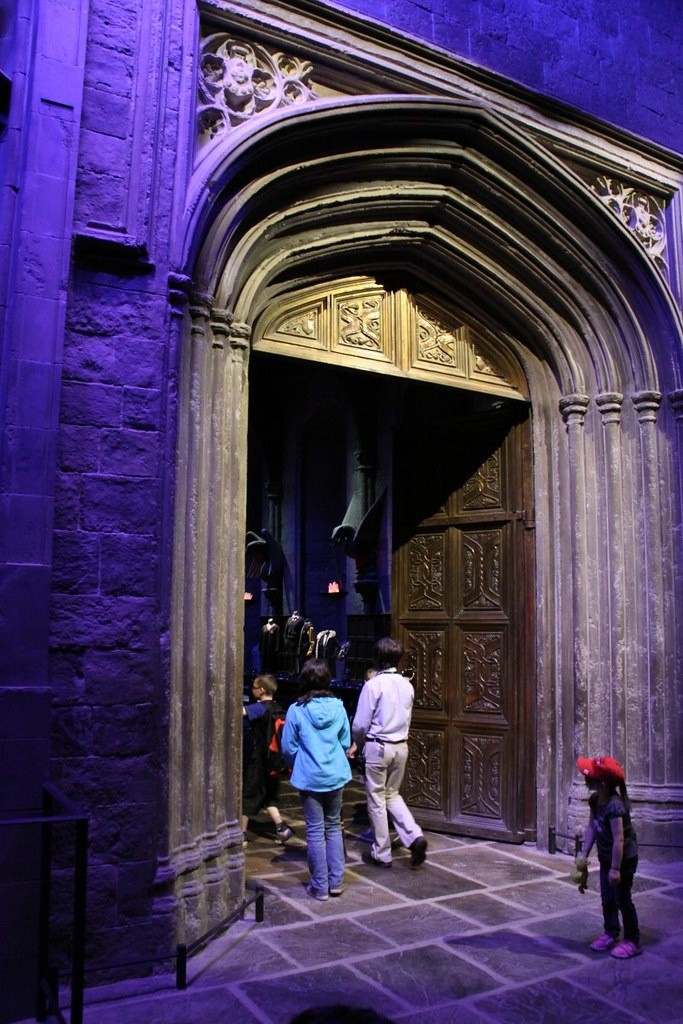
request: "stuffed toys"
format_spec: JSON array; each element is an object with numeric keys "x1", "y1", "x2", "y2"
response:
[{"x1": 570, "y1": 856, "x2": 591, "y2": 894}]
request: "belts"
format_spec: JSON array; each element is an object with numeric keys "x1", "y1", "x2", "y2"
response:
[{"x1": 365, "y1": 738, "x2": 405, "y2": 745}]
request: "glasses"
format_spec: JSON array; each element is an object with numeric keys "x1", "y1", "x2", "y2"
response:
[{"x1": 248, "y1": 682, "x2": 260, "y2": 690}]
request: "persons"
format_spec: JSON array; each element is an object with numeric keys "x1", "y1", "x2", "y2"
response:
[
  {"x1": 258, "y1": 618, "x2": 280, "y2": 674},
  {"x1": 283, "y1": 610, "x2": 315, "y2": 681},
  {"x1": 243, "y1": 675, "x2": 293, "y2": 849},
  {"x1": 281, "y1": 658, "x2": 352, "y2": 901},
  {"x1": 316, "y1": 630, "x2": 341, "y2": 684},
  {"x1": 348, "y1": 637, "x2": 428, "y2": 869},
  {"x1": 574, "y1": 756, "x2": 644, "y2": 959}
]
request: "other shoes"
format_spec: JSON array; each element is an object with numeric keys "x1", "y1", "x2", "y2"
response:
[
  {"x1": 242, "y1": 832, "x2": 248, "y2": 848},
  {"x1": 306, "y1": 882, "x2": 329, "y2": 901},
  {"x1": 407, "y1": 837, "x2": 427, "y2": 869},
  {"x1": 275, "y1": 822, "x2": 292, "y2": 845},
  {"x1": 330, "y1": 883, "x2": 345, "y2": 893},
  {"x1": 391, "y1": 838, "x2": 404, "y2": 851},
  {"x1": 362, "y1": 851, "x2": 392, "y2": 868},
  {"x1": 360, "y1": 826, "x2": 375, "y2": 842}
]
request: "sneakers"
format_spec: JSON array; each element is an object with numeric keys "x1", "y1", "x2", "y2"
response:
[
  {"x1": 589, "y1": 933, "x2": 621, "y2": 952},
  {"x1": 610, "y1": 939, "x2": 643, "y2": 959}
]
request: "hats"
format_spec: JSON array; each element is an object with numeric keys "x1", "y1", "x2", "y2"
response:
[{"x1": 578, "y1": 756, "x2": 625, "y2": 784}]
